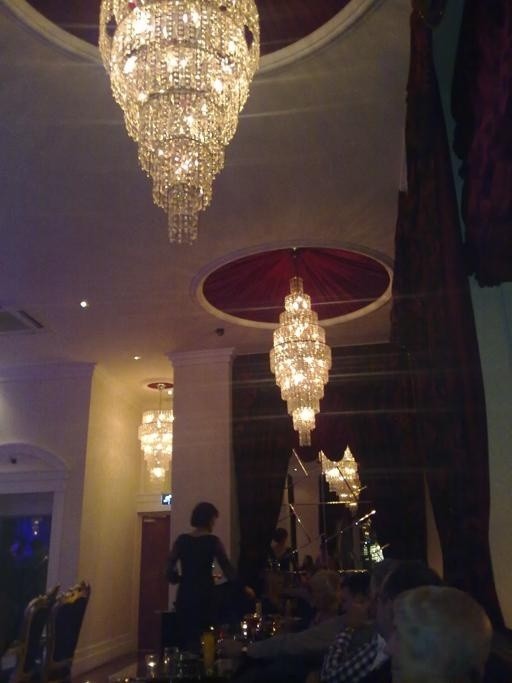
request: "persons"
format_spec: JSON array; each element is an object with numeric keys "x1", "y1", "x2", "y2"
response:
[
  {"x1": 163, "y1": 502, "x2": 256, "y2": 649},
  {"x1": 299, "y1": 555, "x2": 314, "y2": 570},
  {"x1": 308, "y1": 569, "x2": 338, "y2": 630},
  {"x1": 271, "y1": 526, "x2": 294, "y2": 571},
  {"x1": 320, "y1": 560, "x2": 493, "y2": 683}
]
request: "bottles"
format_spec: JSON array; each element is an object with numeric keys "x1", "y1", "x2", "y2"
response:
[{"x1": 213, "y1": 639, "x2": 227, "y2": 682}]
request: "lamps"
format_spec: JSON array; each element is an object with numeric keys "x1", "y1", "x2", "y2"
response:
[
  {"x1": 270, "y1": 244, "x2": 338, "y2": 448},
  {"x1": 137, "y1": 380, "x2": 180, "y2": 481},
  {"x1": 96, "y1": 0, "x2": 259, "y2": 249}
]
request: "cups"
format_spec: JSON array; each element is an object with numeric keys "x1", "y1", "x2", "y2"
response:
[{"x1": 144, "y1": 632, "x2": 213, "y2": 683}]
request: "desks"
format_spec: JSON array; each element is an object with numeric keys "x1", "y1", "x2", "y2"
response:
[{"x1": 107, "y1": 640, "x2": 311, "y2": 681}]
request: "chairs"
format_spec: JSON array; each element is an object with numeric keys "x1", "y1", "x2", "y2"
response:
[{"x1": 0, "y1": 582, "x2": 93, "y2": 682}]
